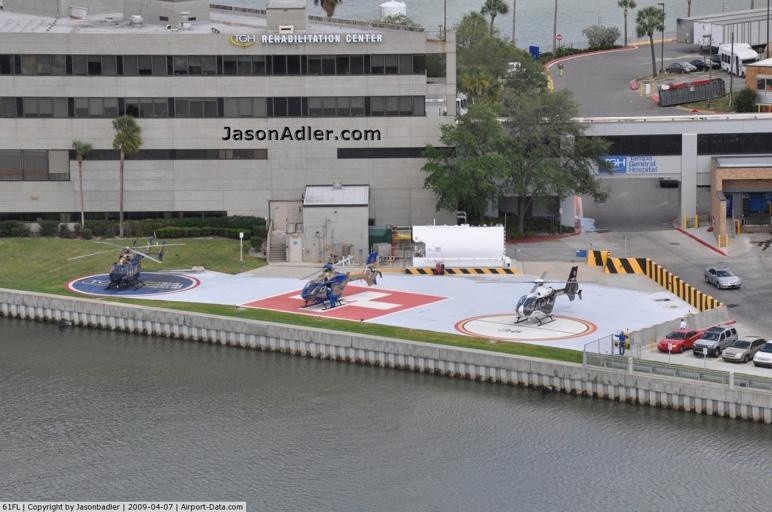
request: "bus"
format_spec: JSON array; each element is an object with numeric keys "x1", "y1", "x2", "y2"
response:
[{"x1": 720, "y1": 42, "x2": 759, "y2": 77}]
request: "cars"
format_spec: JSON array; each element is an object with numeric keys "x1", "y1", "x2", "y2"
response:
[
  {"x1": 753, "y1": 340, "x2": 772, "y2": 367},
  {"x1": 706, "y1": 265, "x2": 741, "y2": 290},
  {"x1": 657, "y1": 329, "x2": 704, "y2": 353},
  {"x1": 721, "y1": 336, "x2": 766, "y2": 363},
  {"x1": 660, "y1": 177, "x2": 677, "y2": 187},
  {"x1": 665, "y1": 58, "x2": 719, "y2": 74}
]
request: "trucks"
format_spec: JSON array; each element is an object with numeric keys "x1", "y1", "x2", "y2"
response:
[{"x1": 506, "y1": 62, "x2": 526, "y2": 73}]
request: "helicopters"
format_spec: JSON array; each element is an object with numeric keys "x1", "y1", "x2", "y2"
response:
[
  {"x1": 476, "y1": 266, "x2": 583, "y2": 326},
  {"x1": 68, "y1": 237, "x2": 186, "y2": 292},
  {"x1": 300, "y1": 252, "x2": 383, "y2": 311}
]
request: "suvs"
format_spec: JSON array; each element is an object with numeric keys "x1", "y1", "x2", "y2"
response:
[{"x1": 693, "y1": 324, "x2": 738, "y2": 357}]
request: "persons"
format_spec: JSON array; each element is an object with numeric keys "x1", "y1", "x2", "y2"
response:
[
  {"x1": 615, "y1": 331, "x2": 627, "y2": 355},
  {"x1": 680, "y1": 318, "x2": 687, "y2": 329}
]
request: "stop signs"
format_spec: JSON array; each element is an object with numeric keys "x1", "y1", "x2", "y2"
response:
[{"x1": 556, "y1": 35, "x2": 562, "y2": 40}]
